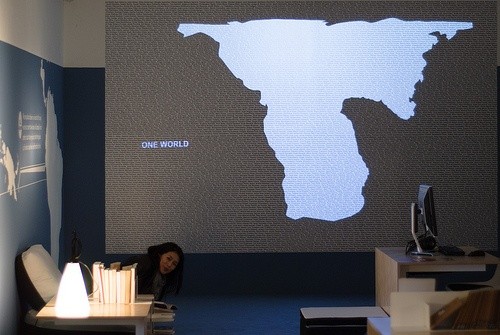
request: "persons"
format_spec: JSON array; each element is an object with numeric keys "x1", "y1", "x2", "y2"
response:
[{"x1": 135, "y1": 242, "x2": 184, "y2": 306}]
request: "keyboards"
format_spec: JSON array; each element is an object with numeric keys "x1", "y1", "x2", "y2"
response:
[{"x1": 439, "y1": 245, "x2": 466, "y2": 255}]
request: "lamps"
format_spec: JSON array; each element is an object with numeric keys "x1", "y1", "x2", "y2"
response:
[{"x1": 55, "y1": 232, "x2": 91, "y2": 319}]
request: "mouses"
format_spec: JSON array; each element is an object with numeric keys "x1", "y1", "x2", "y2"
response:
[{"x1": 469, "y1": 250, "x2": 485, "y2": 256}]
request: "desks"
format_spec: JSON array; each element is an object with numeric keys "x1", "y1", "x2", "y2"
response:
[
  {"x1": 300, "y1": 306, "x2": 390, "y2": 335},
  {"x1": 375, "y1": 247, "x2": 500, "y2": 317},
  {"x1": 36, "y1": 293, "x2": 152, "y2": 335}
]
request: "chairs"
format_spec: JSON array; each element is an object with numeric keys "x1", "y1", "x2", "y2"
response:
[{"x1": 16, "y1": 245, "x2": 62, "y2": 326}]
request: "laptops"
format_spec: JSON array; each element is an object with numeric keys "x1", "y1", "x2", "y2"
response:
[{"x1": 410, "y1": 184, "x2": 437, "y2": 256}]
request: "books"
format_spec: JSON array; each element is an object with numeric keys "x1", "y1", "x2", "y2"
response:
[
  {"x1": 151, "y1": 302, "x2": 178, "y2": 335},
  {"x1": 91, "y1": 261, "x2": 138, "y2": 303}
]
